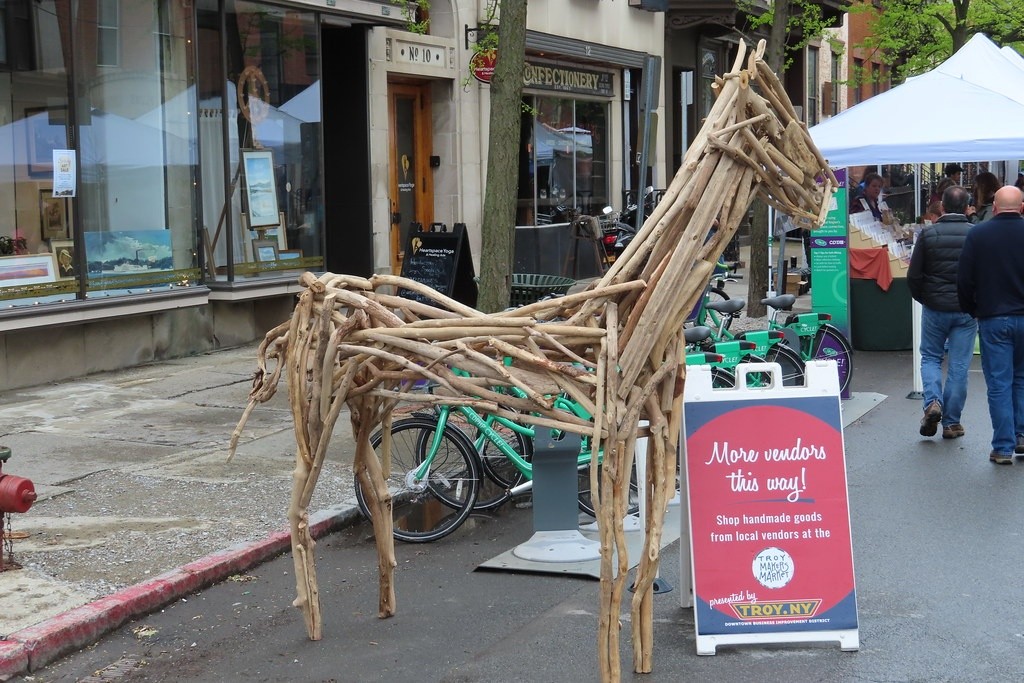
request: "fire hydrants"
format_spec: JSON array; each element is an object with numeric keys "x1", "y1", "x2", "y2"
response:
[{"x1": 0, "y1": 444, "x2": 37, "y2": 575}]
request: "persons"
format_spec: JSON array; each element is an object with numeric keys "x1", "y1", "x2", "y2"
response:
[
  {"x1": 908, "y1": 183, "x2": 977, "y2": 439},
  {"x1": 851, "y1": 173, "x2": 884, "y2": 222},
  {"x1": 945, "y1": 165, "x2": 962, "y2": 182},
  {"x1": 958, "y1": 184, "x2": 1024, "y2": 464},
  {"x1": 927, "y1": 199, "x2": 944, "y2": 223},
  {"x1": 968, "y1": 172, "x2": 1001, "y2": 226}
]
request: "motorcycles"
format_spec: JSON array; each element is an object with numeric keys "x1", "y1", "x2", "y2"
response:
[{"x1": 598, "y1": 186, "x2": 655, "y2": 268}]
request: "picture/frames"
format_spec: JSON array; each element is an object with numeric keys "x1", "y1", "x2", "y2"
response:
[
  {"x1": 49, "y1": 238, "x2": 79, "y2": 282},
  {"x1": 38, "y1": 188, "x2": 71, "y2": 242},
  {"x1": 277, "y1": 249, "x2": 303, "y2": 260},
  {"x1": 252, "y1": 240, "x2": 279, "y2": 261},
  {"x1": 23, "y1": 105, "x2": 73, "y2": 177},
  {"x1": 240, "y1": 148, "x2": 282, "y2": 232}
]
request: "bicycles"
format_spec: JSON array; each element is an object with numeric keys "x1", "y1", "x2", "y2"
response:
[{"x1": 348, "y1": 261, "x2": 856, "y2": 543}]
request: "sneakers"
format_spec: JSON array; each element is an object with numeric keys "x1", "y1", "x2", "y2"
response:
[
  {"x1": 943, "y1": 423, "x2": 964, "y2": 439},
  {"x1": 1014, "y1": 434, "x2": 1024, "y2": 454},
  {"x1": 990, "y1": 449, "x2": 1013, "y2": 464},
  {"x1": 919, "y1": 401, "x2": 941, "y2": 436}
]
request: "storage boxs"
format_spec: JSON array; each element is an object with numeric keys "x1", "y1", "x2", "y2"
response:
[{"x1": 847, "y1": 211, "x2": 911, "y2": 281}]
